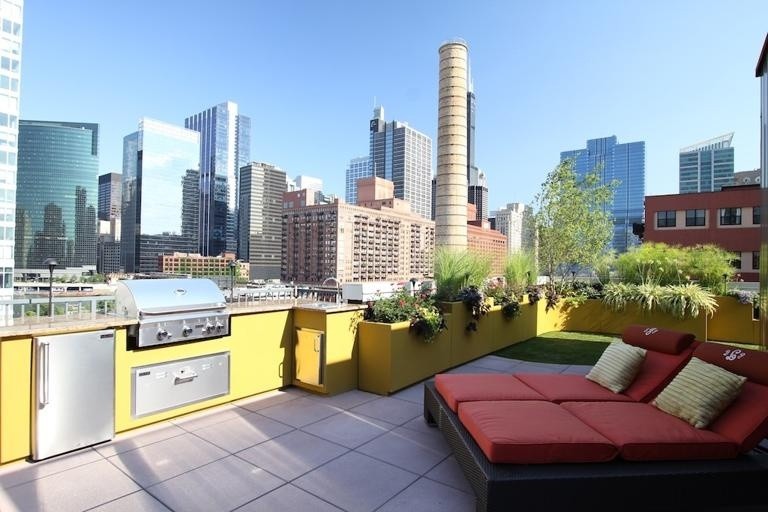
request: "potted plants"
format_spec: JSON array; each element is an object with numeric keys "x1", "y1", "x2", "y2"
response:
[{"x1": 358, "y1": 154, "x2": 768, "y2": 396}]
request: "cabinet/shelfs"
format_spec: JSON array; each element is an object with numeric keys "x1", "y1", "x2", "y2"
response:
[{"x1": 291, "y1": 326, "x2": 325, "y2": 389}]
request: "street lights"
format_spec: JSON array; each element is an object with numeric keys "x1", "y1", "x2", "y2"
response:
[
  {"x1": 409, "y1": 278, "x2": 418, "y2": 293},
  {"x1": 229, "y1": 262, "x2": 236, "y2": 294},
  {"x1": 42, "y1": 257, "x2": 61, "y2": 303}
]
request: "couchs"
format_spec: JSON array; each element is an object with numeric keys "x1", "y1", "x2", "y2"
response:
[{"x1": 422, "y1": 325, "x2": 768, "y2": 512}]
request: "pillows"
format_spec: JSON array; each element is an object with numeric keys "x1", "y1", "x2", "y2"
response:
[
  {"x1": 650, "y1": 356, "x2": 748, "y2": 430},
  {"x1": 584, "y1": 335, "x2": 648, "y2": 396}
]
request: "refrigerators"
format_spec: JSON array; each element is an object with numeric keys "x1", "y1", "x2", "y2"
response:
[{"x1": 32, "y1": 329, "x2": 116, "y2": 463}]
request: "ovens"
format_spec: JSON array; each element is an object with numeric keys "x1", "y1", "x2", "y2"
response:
[{"x1": 130, "y1": 353, "x2": 231, "y2": 417}]
